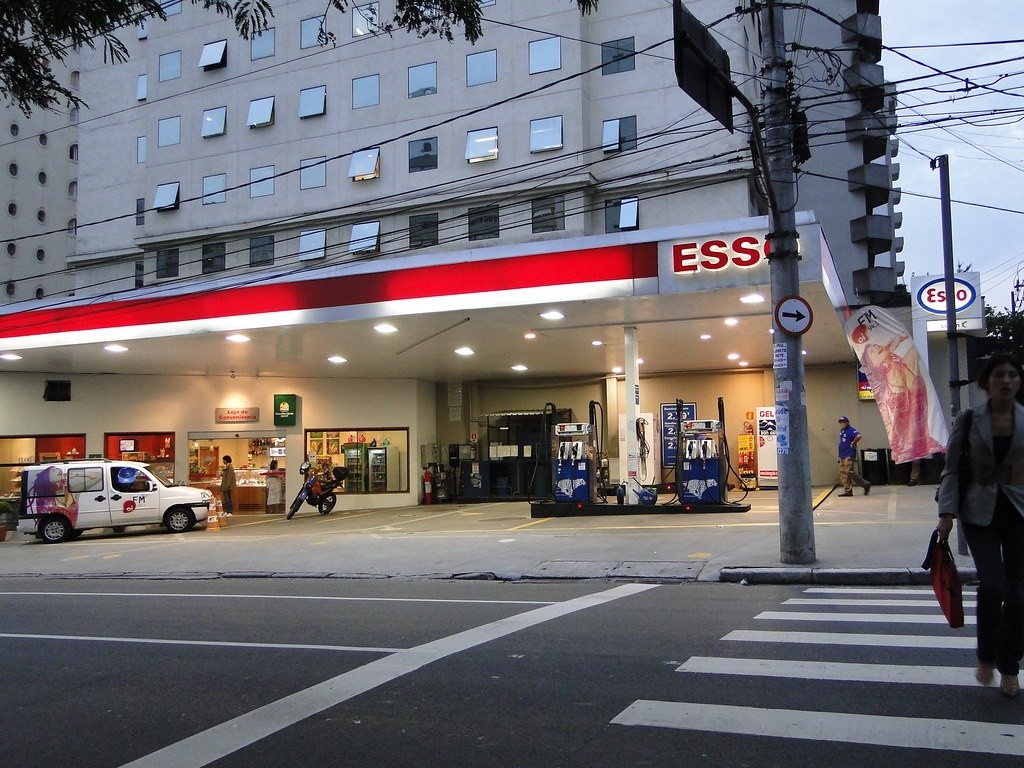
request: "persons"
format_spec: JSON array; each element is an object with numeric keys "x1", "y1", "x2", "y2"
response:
[
  {"x1": 838, "y1": 416, "x2": 871, "y2": 497},
  {"x1": 27, "y1": 466, "x2": 103, "y2": 529},
  {"x1": 221, "y1": 455, "x2": 236, "y2": 517},
  {"x1": 266, "y1": 460, "x2": 283, "y2": 513},
  {"x1": 907, "y1": 458, "x2": 921, "y2": 486},
  {"x1": 937, "y1": 354, "x2": 1024, "y2": 697},
  {"x1": 860, "y1": 330, "x2": 944, "y2": 464}
]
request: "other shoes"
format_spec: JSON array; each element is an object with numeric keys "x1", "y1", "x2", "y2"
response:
[
  {"x1": 226, "y1": 512, "x2": 234, "y2": 518},
  {"x1": 1000, "y1": 674, "x2": 1020, "y2": 699},
  {"x1": 864, "y1": 482, "x2": 872, "y2": 496},
  {"x1": 976, "y1": 665, "x2": 994, "y2": 686},
  {"x1": 217, "y1": 512, "x2": 228, "y2": 518},
  {"x1": 907, "y1": 481, "x2": 918, "y2": 486},
  {"x1": 838, "y1": 493, "x2": 853, "y2": 497}
]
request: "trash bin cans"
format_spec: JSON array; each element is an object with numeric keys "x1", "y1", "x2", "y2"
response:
[
  {"x1": 860, "y1": 448, "x2": 888, "y2": 485},
  {"x1": 888, "y1": 448, "x2": 913, "y2": 485}
]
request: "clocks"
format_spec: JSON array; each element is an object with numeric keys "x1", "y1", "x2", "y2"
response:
[{"x1": 119, "y1": 438, "x2": 135, "y2": 452}]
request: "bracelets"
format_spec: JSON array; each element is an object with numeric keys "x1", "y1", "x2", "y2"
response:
[{"x1": 898, "y1": 334, "x2": 904, "y2": 342}]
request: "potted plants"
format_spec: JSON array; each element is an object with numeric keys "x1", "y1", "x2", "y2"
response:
[{"x1": 0, "y1": 501, "x2": 13, "y2": 542}]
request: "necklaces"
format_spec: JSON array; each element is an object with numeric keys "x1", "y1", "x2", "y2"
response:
[
  {"x1": 55, "y1": 488, "x2": 68, "y2": 507},
  {"x1": 884, "y1": 362, "x2": 910, "y2": 412}
]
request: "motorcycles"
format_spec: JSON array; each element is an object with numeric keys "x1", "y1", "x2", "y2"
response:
[{"x1": 287, "y1": 462, "x2": 343, "y2": 520}]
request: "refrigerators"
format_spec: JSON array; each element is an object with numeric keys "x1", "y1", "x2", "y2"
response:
[
  {"x1": 368, "y1": 447, "x2": 399, "y2": 492},
  {"x1": 345, "y1": 442, "x2": 370, "y2": 492}
]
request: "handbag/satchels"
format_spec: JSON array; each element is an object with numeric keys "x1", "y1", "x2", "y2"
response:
[
  {"x1": 921, "y1": 530, "x2": 964, "y2": 629},
  {"x1": 935, "y1": 410, "x2": 973, "y2": 519}
]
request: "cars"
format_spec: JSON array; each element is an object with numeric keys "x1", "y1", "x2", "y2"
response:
[{"x1": 16, "y1": 459, "x2": 214, "y2": 543}]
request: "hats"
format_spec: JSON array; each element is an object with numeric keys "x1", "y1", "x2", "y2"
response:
[{"x1": 838, "y1": 416, "x2": 849, "y2": 422}]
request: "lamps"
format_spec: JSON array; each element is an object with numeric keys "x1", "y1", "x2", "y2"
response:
[{"x1": 209, "y1": 440, "x2": 214, "y2": 451}]
